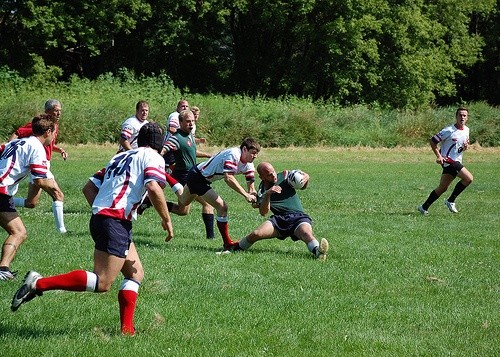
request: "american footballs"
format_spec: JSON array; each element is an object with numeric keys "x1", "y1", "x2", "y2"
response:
[{"x1": 287, "y1": 169, "x2": 309, "y2": 191}]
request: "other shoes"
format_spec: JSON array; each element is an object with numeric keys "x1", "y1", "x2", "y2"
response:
[
  {"x1": 137, "y1": 195, "x2": 152, "y2": 215},
  {"x1": 224, "y1": 241, "x2": 239, "y2": 251}
]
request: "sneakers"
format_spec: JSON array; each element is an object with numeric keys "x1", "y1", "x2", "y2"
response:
[
  {"x1": 316, "y1": 237, "x2": 329, "y2": 261},
  {"x1": 417, "y1": 204, "x2": 429, "y2": 217},
  {"x1": 11, "y1": 270, "x2": 43, "y2": 312},
  {"x1": 444, "y1": 199, "x2": 458, "y2": 213},
  {"x1": 0, "y1": 270, "x2": 19, "y2": 281}
]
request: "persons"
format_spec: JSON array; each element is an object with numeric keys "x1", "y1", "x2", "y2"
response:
[
  {"x1": 216, "y1": 162, "x2": 329, "y2": 260},
  {"x1": 9, "y1": 99, "x2": 68, "y2": 233},
  {"x1": 138, "y1": 138, "x2": 260, "y2": 250},
  {"x1": 117, "y1": 100, "x2": 212, "y2": 174},
  {"x1": 12, "y1": 123, "x2": 174, "y2": 336},
  {"x1": 418, "y1": 107, "x2": 474, "y2": 215},
  {"x1": 161, "y1": 110, "x2": 240, "y2": 249},
  {"x1": 0, "y1": 114, "x2": 59, "y2": 281}
]
